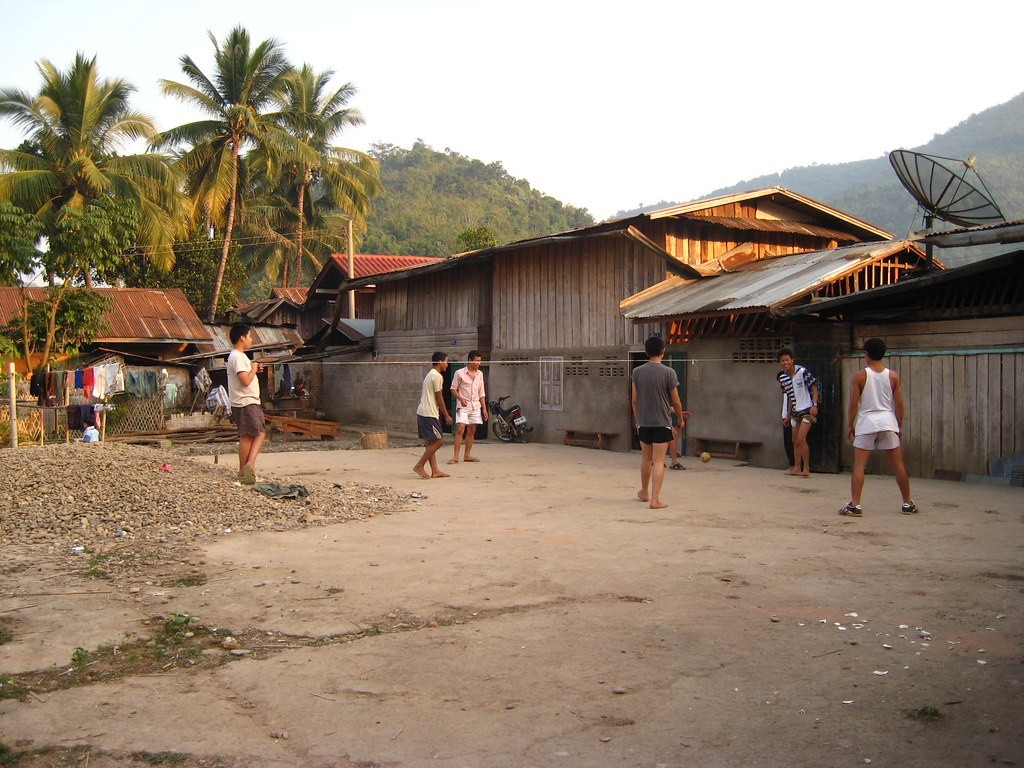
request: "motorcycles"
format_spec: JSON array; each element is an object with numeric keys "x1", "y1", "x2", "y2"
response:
[{"x1": 490, "y1": 396, "x2": 534, "y2": 443}]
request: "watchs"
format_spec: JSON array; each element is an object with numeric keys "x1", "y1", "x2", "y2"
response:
[{"x1": 813, "y1": 402, "x2": 818, "y2": 407}]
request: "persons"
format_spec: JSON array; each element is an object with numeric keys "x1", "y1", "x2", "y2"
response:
[
  {"x1": 632, "y1": 337, "x2": 685, "y2": 509},
  {"x1": 776, "y1": 347, "x2": 819, "y2": 476},
  {"x1": 78, "y1": 418, "x2": 100, "y2": 443},
  {"x1": 413, "y1": 352, "x2": 453, "y2": 478},
  {"x1": 227, "y1": 324, "x2": 266, "y2": 484},
  {"x1": 837, "y1": 338, "x2": 919, "y2": 517},
  {"x1": 448, "y1": 350, "x2": 488, "y2": 464},
  {"x1": 651, "y1": 407, "x2": 692, "y2": 470}
]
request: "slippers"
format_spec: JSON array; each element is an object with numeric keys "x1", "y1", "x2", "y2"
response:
[
  {"x1": 652, "y1": 463, "x2": 666, "y2": 467},
  {"x1": 446, "y1": 460, "x2": 459, "y2": 464},
  {"x1": 670, "y1": 463, "x2": 686, "y2": 470},
  {"x1": 463, "y1": 457, "x2": 480, "y2": 462}
]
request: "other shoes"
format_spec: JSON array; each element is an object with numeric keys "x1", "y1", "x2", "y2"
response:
[{"x1": 242, "y1": 464, "x2": 256, "y2": 485}]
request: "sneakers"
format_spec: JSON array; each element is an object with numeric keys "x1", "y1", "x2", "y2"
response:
[
  {"x1": 838, "y1": 500, "x2": 863, "y2": 517},
  {"x1": 901, "y1": 500, "x2": 918, "y2": 515}
]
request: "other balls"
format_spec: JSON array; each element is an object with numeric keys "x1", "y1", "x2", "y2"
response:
[{"x1": 701, "y1": 452, "x2": 711, "y2": 463}]
